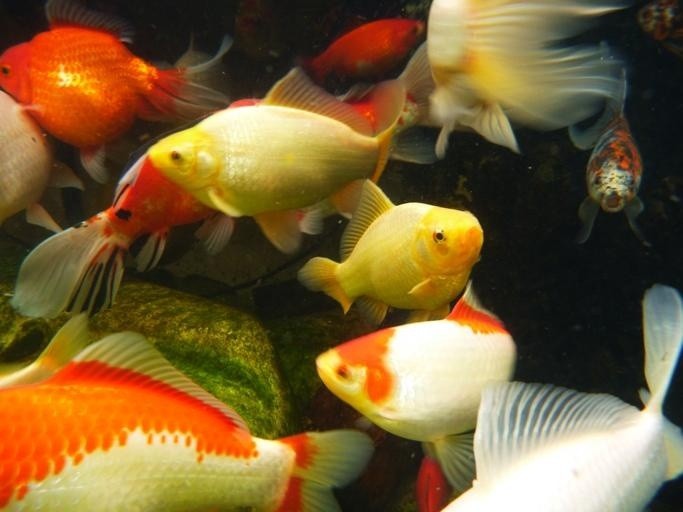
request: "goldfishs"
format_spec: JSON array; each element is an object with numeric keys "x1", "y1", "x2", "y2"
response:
[{"x1": 0, "y1": 0, "x2": 683, "y2": 512}]
